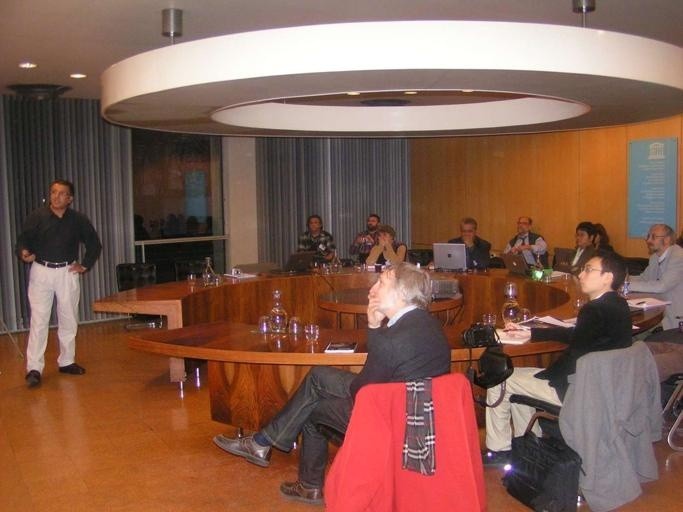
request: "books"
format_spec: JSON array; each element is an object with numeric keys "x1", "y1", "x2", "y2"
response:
[{"x1": 324, "y1": 341, "x2": 358, "y2": 353}]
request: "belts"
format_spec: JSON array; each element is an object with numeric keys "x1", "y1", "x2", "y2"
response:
[{"x1": 32, "y1": 257, "x2": 75, "y2": 270}]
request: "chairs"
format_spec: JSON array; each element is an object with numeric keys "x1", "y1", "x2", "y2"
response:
[
  {"x1": 114, "y1": 262, "x2": 165, "y2": 330},
  {"x1": 287, "y1": 242, "x2": 650, "y2": 277},
  {"x1": 177, "y1": 258, "x2": 206, "y2": 280},
  {"x1": 508, "y1": 344, "x2": 662, "y2": 508},
  {"x1": 322, "y1": 372, "x2": 484, "y2": 512}
]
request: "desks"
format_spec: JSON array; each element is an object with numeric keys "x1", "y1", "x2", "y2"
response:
[{"x1": 91, "y1": 265, "x2": 666, "y2": 435}]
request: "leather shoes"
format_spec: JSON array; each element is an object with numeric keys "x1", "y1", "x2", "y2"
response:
[
  {"x1": 25, "y1": 370, "x2": 40, "y2": 384},
  {"x1": 279, "y1": 480, "x2": 324, "y2": 504},
  {"x1": 212, "y1": 431, "x2": 273, "y2": 470},
  {"x1": 57, "y1": 363, "x2": 86, "y2": 375},
  {"x1": 479, "y1": 448, "x2": 510, "y2": 466}
]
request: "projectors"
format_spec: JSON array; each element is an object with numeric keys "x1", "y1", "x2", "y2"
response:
[{"x1": 430, "y1": 277, "x2": 459, "y2": 300}]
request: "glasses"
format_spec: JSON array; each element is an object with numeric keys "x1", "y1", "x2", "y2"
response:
[
  {"x1": 517, "y1": 221, "x2": 528, "y2": 226},
  {"x1": 579, "y1": 265, "x2": 605, "y2": 276},
  {"x1": 644, "y1": 233, "x2": 664, "y2": 240}
]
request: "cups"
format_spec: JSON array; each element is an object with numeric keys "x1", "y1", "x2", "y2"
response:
[
  {"x1": 187, "y1": 274, "x2": 196, "y2": 284},
  {"x1": 519, "y1": 308, "x2": 529, "y2": 320},
  {"x1": 309, "y1": 261, "x2": 342, "y2": 274},
  {"x1": 308, "y1": 342, "x2": 318, "y2": 353},
  {"x1": 258, "y1": 315, "x2": 271, "y2": 332},
  {"x1": 374, "y1": 264, "x2": 380, "y2": 272},
  {"x1": 304, "y1": 325, "x2": 319, "y2": 341},
  {"x1": 231, "y1": 265, "x2": 240, "y2": 276},
  {"x1": 289, "y1": 335, "x2": 302, "y2": 345},
  {"x1": 480, "y1": 312, "x2": 496, "y2": 326},
  {"x1": 258, "y1": 331, "x2": 270, "y2": 344},
  {"x1": 288, "y1": 317, "x2": 302, "y2": 336}
]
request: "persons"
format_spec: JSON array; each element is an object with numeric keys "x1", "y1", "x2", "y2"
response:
[
  {"x1": 554, "y1": 221, "x2": 613, "y2": 275},
  {"x1": 617, "y1": 224, "x2": 682, "y2": 383},
  {"x1": 349, "y1": 216, "x2": 407, "y2": 272},
  {"x1": 298, "y1": 216, "x2": 337, "y2": 268},
  {"x1": 503, "y1": 216, "x2": 547, "y2": 269},
  {"x1": 15, "y1": 180, "x2": 102, "y2": 384},
  {"x1": 428, "y1": 217, "x2": 491, "y2": 271},
  {"x1": 214, "y1": 262, "x2": 452, "y2": 502},
  {"x1": 481, "y1": 249, "x2": 632, "y2": 466}
]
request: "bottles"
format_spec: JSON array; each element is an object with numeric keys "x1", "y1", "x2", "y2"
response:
[
  {"x1": 200, "y1": 257, "x2": 216, "y2": 286},
  {"x1": 534, "y1": 248, "x2": 543, "y2": 272},
  {"x1": 270, "y1": 290, "x2": 289, "y2": 331},
  {"x1": 270, "y1": 331, "x2": 289, "y2": 352},
  {"x1": 501, "y1": 282, "x2": 521, "y2": 332},
  {"x1": 622, "y1": 271, "x2": 631, "y2": 300}
]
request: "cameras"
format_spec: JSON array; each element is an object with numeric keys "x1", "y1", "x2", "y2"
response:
[{"x1": 461, "y1": 323, "x2": 494, "y2": 346}]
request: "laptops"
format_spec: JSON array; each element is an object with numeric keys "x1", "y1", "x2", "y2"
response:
[
  {"x1": 501, "y1": 254, "x2": 531, "y2": 275},
  {"x1": 270, "y1": 251, "x2": 313, "y2": 274},
  {"x1": 431, "y1": 242, "x2": 466, "y2": 272}
]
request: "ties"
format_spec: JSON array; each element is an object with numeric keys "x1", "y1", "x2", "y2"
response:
[{"x1": 520, "y1": 237, "x2": 526, "y2": 253}]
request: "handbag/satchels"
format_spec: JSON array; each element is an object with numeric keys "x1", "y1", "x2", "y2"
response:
[{"x1": 501, "y1": 412, "x2": 585, "y2": 512}]
